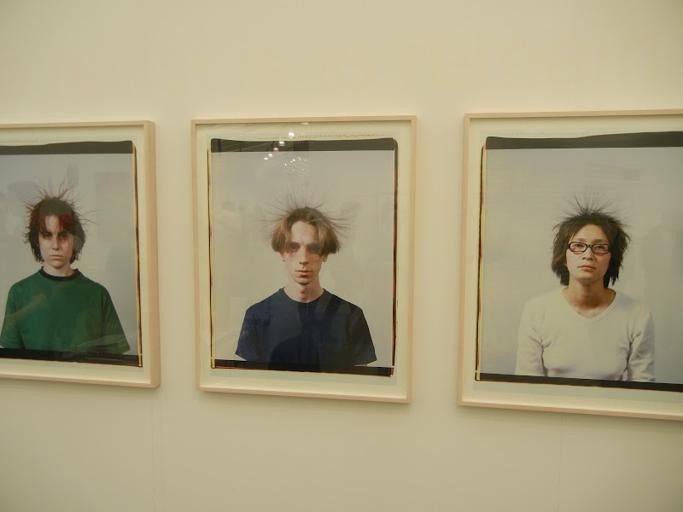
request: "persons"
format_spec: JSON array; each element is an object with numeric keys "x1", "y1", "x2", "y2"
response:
[
  {"x1": 514, "y1": 212, "x2": 656, "y2": 382},
  {"x1": 0, "y1": 197, "x2": 130, "y2": 354},
  {"x1": 235, "y1": 206, "x2": 376, "y2": 366}
]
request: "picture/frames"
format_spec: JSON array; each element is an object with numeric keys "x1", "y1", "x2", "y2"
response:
[
  {"x1": 0, "y1": 120, "x2": 160, "y2": 389},
  {"x1": 458, "y1": 107, "x2": 683, "y2": 422},
  {"x1": 190, "y1": 116, "x2": 416, "y2": 404}
]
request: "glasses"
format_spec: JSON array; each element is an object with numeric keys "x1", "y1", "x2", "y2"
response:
[{"x1": 567, "y1": 241, "x2": 610, "y2": 256}]
